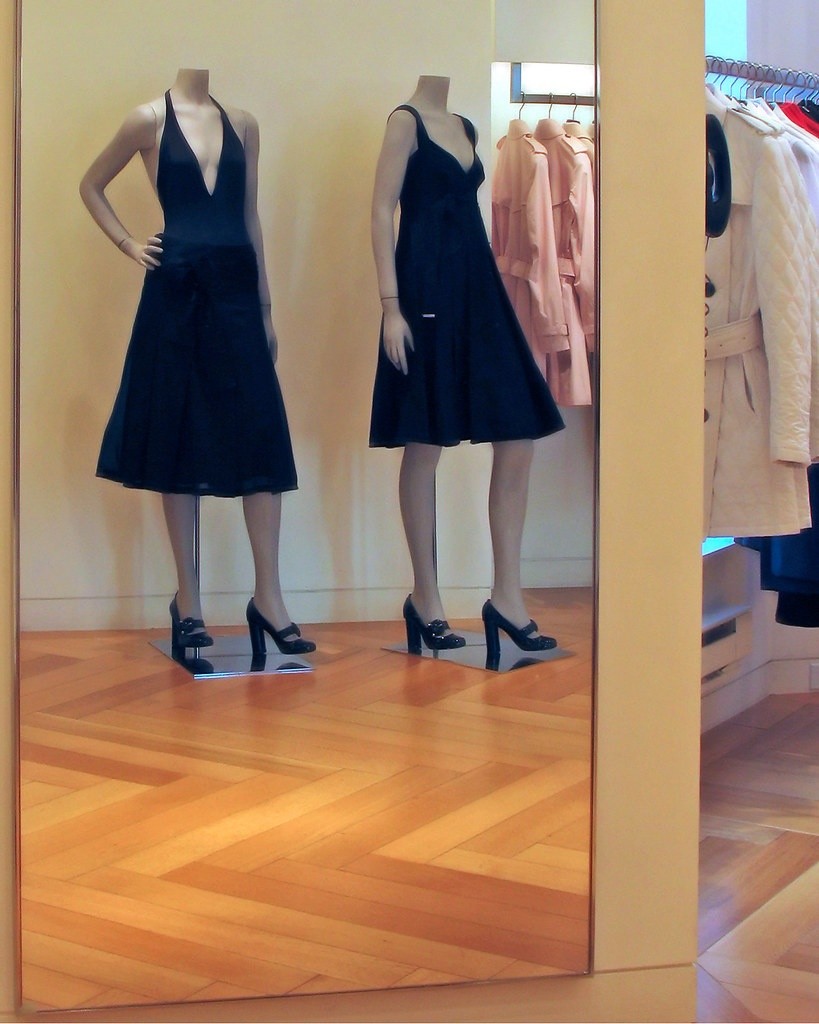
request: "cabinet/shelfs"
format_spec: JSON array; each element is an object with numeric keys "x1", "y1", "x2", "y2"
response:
[{"x1": 701, "y1": 537, "x2": 818, "y2": 734}]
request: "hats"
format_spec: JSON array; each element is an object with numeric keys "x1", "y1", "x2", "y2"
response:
[{"x1": 706, "y1": 112, "x2": 734, "y2": 238}]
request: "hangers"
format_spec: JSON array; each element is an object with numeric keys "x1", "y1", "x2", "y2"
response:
[
  {"x1": 518, "y1": 91, "x2": 578, "y2": 121},
  {"x1": 705, "y1": 55, "x2": 819, "y2": 113}
]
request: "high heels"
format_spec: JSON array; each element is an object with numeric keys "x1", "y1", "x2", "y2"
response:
[
  {"x1": 402, "y1": 593, "x2": 467, "y2": 654},
  {"x1": 480, "y1": 599, "x2": 558, "y2": 652},
  {"x1": 168, "y1": 590, "x2": 215, "y2": 648},
  {"x1": 245, "y1": 596, "x2": 316, "y2": 654}
]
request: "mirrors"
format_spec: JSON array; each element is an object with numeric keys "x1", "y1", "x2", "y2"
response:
[{"x1": 14, "y1": 0, "x2": 598, "y2": 1013}]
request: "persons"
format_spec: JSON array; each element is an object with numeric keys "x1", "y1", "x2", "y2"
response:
[
  {"x1": 80, "y1": 68, "x2": 317, "y2": 656},
  {"x1": 367, "y1": 74, "x2": 561, "y2": 654}
]
